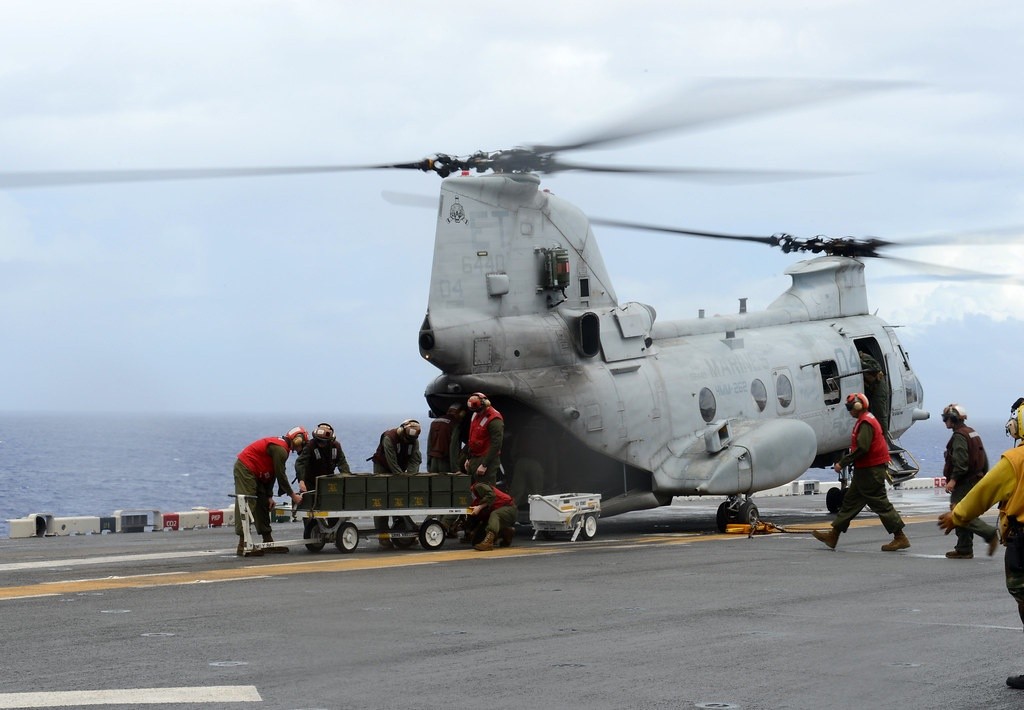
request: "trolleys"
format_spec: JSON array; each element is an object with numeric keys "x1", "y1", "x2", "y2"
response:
[{"x1": 528, "y1": 493, "x2": 601, "y2": 542}]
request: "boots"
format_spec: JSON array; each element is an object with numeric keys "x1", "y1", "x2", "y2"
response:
[
  {"x1": 500, "y1": 526, "x2": 515, "y2": 548},
  {"x1": 261, "y1": 532, "x2": 289, "y2": 553},
  {"x1": 812, "y1": 526, "x2": 841, "y2": 548},
  {"x1": 237, "y1": 535, "x2": 264, "y2": 556},
  {"x1": 475, "y1": 531, "x2": 495, "y2": 551},
  {"x1": 882, "y1": 529, "x2": 910, "y2": 551}
]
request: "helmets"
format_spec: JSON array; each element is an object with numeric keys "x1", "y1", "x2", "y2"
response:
[
  {"x1": 447, "y1": 402, "x2": 468, "y2": 422},
  {"x1": 467, "y1": 392, "x2": 491, "y2": 415},
  {"x1": 944, "y1": 405, "x2": 968, "y2": 420},
  {"x1": 313, "y1": 423, "x2": 336, "y2": 443},
  {"x1": 396, "y1": 420, "x2": 421, "y2": 444},
  {"x1": 1007, "y1": 398, "x2": 1024, "y2": 439},
  {"x1": 847, "y1": 393, "x2": 869, "y2": 418},
  {"x1": 286, "y1": 426, "x2": 310, "y2": 453}
]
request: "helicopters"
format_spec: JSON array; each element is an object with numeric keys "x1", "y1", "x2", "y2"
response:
[{"x1": 0, "y1": 73, "x2": 1024, "y2": 535}]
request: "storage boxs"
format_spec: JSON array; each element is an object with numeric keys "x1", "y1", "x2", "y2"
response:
[
  {"x1": 292, "y1": 473, "x2": 473, "y2": 511},
  {"x1": 527, "y1": 492, "x2": 602, "y2": 522}
]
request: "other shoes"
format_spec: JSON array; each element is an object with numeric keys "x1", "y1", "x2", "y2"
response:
[
  {"x1": 379, "y1": 537, "x2": 392, "y2": 548},
  {"x1": 460, "y1": 532, "x2": 474, "y2": 543},
  {"x1": 411, "y1": 539, "x2": 419, "y2": 545},
  {"x1": 1006, "y1": 675, "x2": 1024, "y2": 689}
]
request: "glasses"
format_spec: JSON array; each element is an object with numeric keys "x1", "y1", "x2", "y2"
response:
[
  {"x1": 845, "y1": 403, "x2": 853, "y2": 411},
  {"x1": 941, "y1": 414, "x2": 948, "y2": 422}
]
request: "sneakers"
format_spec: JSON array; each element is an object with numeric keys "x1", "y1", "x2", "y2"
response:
[
  {"x1": 945, "y1": 550, "x2": 973, "y2": 559},
  {"x1": 988, "y1": 529, "x2": 999, "y2": 556}
]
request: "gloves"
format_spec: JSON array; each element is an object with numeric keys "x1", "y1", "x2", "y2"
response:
[{"x1": 938, "y1": 512, "x2": 958, "y2": 535}]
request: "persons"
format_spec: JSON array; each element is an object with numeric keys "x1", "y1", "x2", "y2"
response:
[
  {"x1": 812, "y1": 392, "x2": 910, "y2": 552},
  {"x1": 233, "y1": 426, "x2": 309, "y2": 556},
  {"x1": 294, "y1": 422, "x2": 350, "y2": 528},
  {"x1": 941, "y1": 403, "x2": 999, "y2": 558},
  {"x1": 858, "y1": 351, "x2": 889, "y2": 439},
  {"x1": 373, "y1": 419, "x2": 422, "y2": 547},
  {"x1": 459, "y1": 392, "x2": 504, "y2": 543},
  {"x1": 469, "y1": 482, "x2": 515, "y2": 552},
  {"x1": 937, "y1": 397, "x2": 1024, "y2": 689},
  {"x1": 427, "y1": 403, "x2": 465, "y2": 538}
]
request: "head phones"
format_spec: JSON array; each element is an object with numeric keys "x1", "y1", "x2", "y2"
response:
[
  {"x1": 854, "y1": 393, "x2": 863, "y2": 410},
  {"x1": 1007, "y1": 398, "x2": 1024, "y2": 439},
  {"x1": 397, "y1": 420, "x2": 419, "y2": 436},
  {"x1": 473, "y1": 393, "x2": 491, "y2": 407},
  {"x1": 293, "y1": 437, "x2": 304, "y2": 447},
  {"x1": 949, "y1": 405, "x2": 958, "y2": 423},
  {"x1": 312, "y1": 423, "x2": 337, "y2": 443}
]
